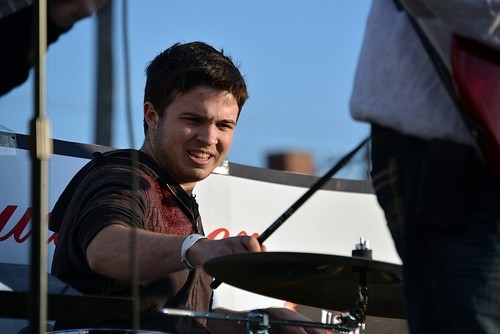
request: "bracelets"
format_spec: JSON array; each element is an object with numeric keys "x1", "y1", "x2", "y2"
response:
[{"x1": 181, "y1": 234, "x2": 206, "y2": 271}]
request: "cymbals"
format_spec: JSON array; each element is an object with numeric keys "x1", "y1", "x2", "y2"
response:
[
  {"x1": 202, "y1": 252, "x2": 406, "y2": 320},
  {"x1": 0, "y1": 290, "x2": 132, "y2": 324}
]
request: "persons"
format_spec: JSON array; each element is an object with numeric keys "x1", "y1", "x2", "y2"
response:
[
  {"x1": 349, "y1": 0, "x2": 500, "y2": 334},
  {"x1": 48, "y1": 41, "x2": 332, "y2": 334}
]
